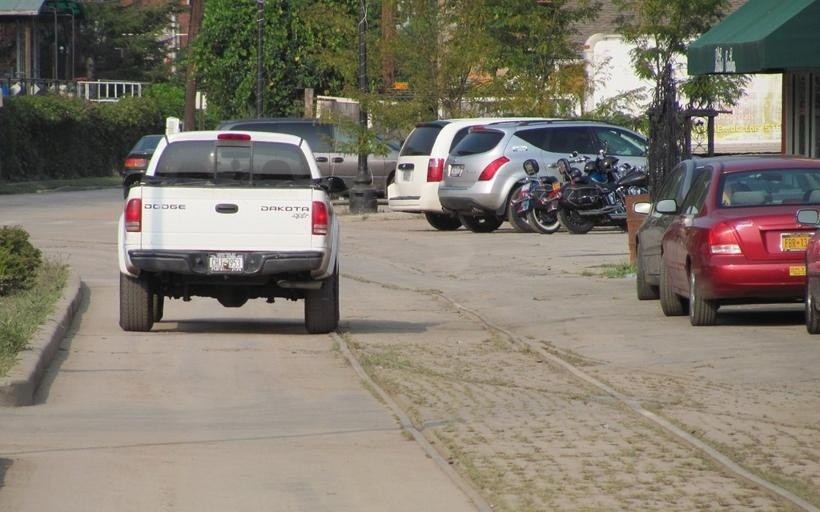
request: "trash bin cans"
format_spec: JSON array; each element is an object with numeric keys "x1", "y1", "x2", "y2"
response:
[{"x1": 625, "y1": 194, "x2": 651, "y2": 263}]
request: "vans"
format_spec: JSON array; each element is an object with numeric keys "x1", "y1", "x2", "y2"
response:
[{"x1": 212, "y1": 116, "x2": 402, "y2": 200}]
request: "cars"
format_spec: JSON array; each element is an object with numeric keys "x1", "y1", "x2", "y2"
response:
[
  {"x1": 795, "y1": 205, "x2": 820, "y2": 333},
  {"x1": 633, "y1": 151, "x2": 802, "y2": 301},
  {"x1": 121, "y1": 134, "x2": 162, "y2": 193},
  {"x1": 654, "y1": 156, "x2": 820, "y2": 327}
]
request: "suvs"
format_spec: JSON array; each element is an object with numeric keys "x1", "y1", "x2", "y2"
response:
[
  {"x1": 435, "y1": 118, "x2": 700, "y2": 231},
  {"x1": 386, "y1": 118, "x2": 570, "y2": 235}
]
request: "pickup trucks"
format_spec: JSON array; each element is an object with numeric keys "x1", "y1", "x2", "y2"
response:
[{"x1": 115, "y1": 128, "x2": 347, "y2": 333}]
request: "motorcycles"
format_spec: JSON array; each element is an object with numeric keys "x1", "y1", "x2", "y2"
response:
[{"x1": 520, "y1": 140, "x2": 653, "y2": 235}]
